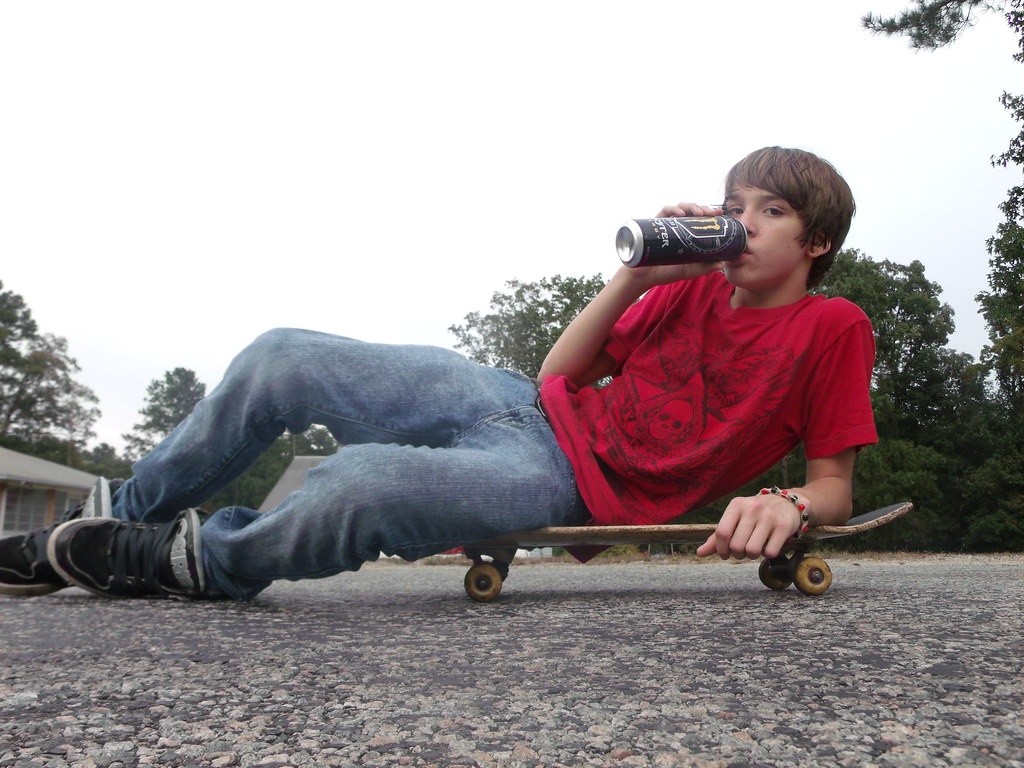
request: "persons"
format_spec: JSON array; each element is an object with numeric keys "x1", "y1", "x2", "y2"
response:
[{"x1": 0, "y1": 144, "x2": 880, "y2": 602}]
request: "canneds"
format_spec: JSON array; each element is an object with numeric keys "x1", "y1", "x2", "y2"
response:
[{"x1": 615, "y1": 215, "x2": 748, "y2": 268}]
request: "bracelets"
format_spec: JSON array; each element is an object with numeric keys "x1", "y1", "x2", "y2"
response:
[{"x1": 755, "y1": 486, "x2": 809, "y2": 539}]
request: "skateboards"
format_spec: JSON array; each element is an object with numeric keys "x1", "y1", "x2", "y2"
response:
[{"x1": 456, "y1": 499, "x2": 914, "y2": 604}]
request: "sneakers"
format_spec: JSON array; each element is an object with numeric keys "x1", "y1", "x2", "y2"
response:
[
  {"x1": 1, "y1": 479, "x2": 122, "y2": 595},
  {"x1": 46, "y1": 508, "x2": 218, "y2": 601}
]
